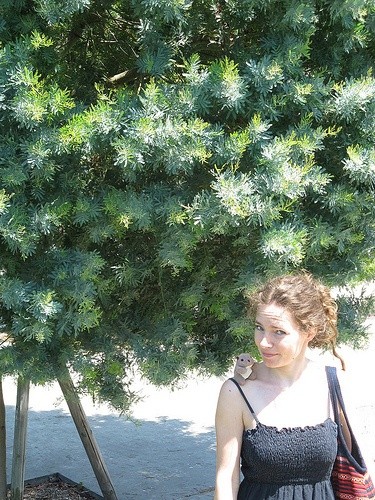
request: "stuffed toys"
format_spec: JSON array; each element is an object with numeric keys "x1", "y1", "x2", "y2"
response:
[{"x1": 233, "y1": 353, "x2": 257, "y2": 385}]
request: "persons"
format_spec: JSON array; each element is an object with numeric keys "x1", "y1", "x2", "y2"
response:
[{"x1": 214, "y1": 274, "x2": 352, "y2": 500}]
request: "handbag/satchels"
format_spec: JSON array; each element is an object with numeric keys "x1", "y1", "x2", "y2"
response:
[{"x1": 325, "y1": 366, "x2": 375, "y2": 500}]
